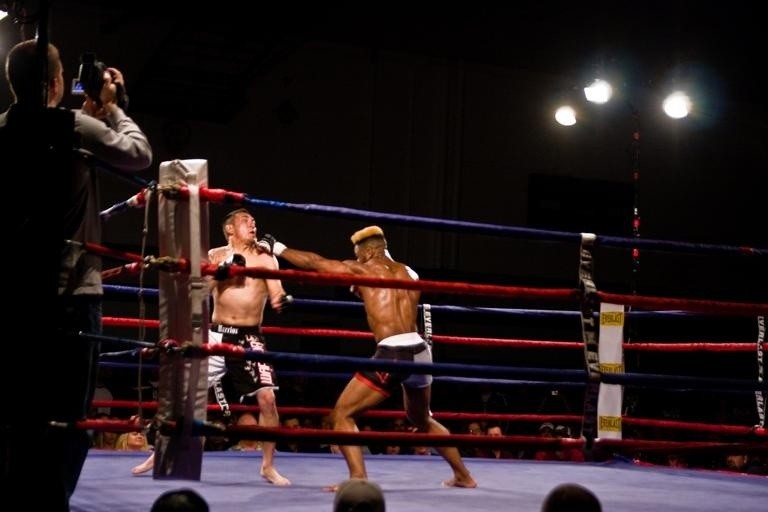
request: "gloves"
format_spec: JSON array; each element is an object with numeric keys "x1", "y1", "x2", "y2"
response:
[
  {"x1": 350, "y1": 285, "x2": 362, "y2": 299},
  {"x1": 256, "y1": 235, "x2": 287, "y2": 256},
  {"x1": 214, "y1": 254, "x2": 246, "y2": 281}
]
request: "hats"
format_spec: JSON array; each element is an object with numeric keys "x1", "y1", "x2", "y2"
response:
[
  {"x1": 540, "y1": 422, "x2": 554, "y2": 431},
  {"x1": 555, "y1": 425, "x2": 574, "y2": 437}
]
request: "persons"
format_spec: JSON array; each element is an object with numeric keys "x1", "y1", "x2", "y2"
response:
[
  {"x1": 255, "y1": 224, "x2": 479, "y2": 492},
  {"x1": 130, "y1": 207, "x2": 296, "y2": 488},
  {"x1": 0, "y1": 38, "x2": 158, "y2": 511},
  {"x1": 84, "y1": 376, "x2": 767, "y2": 480},
  {"x1": 539, "y1": 480, "x2": 602, "y2": 511},
  {"x1": 150, "y1": 487, "x2": 210, "y2": 511}
]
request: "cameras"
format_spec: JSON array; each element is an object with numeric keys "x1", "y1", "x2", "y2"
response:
[{"x1": 70, "y1": 52, "x2": 130, "y2": 109}]
